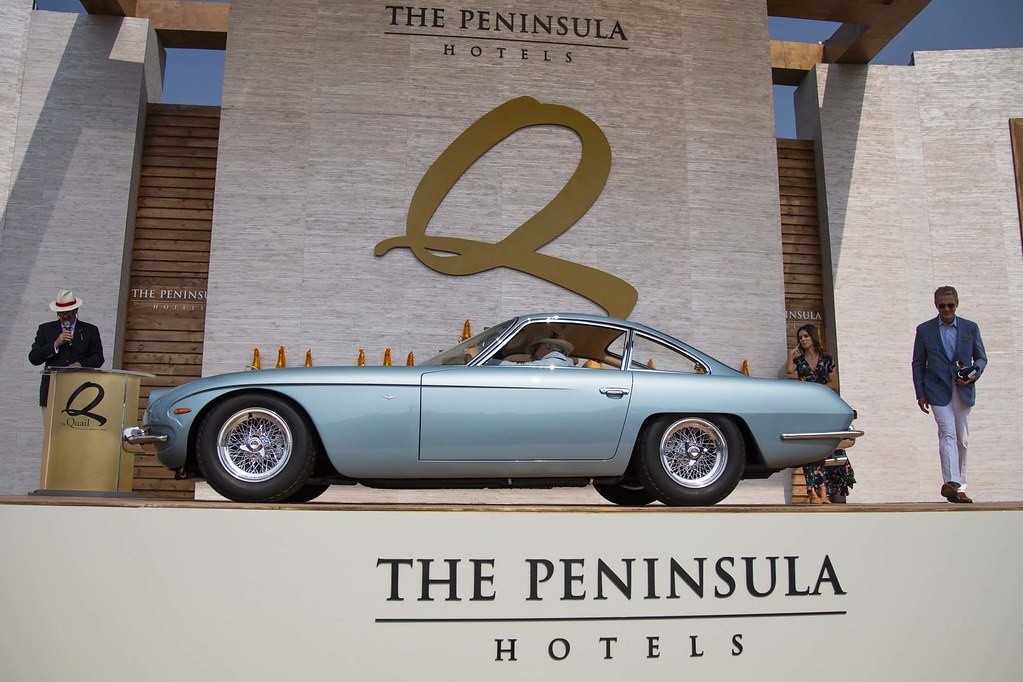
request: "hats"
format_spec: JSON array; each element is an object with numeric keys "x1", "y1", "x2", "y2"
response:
[
  {"x1": 525, "y1": 326, "x2": 575, "y2": 355},
  {"x1": 49, "y1": 289, "x2": 83, "y2": 312}
]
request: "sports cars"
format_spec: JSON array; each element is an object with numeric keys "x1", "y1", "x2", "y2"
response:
[{"x1": 116, "y1": 312, "x2": 865, "y2": 507}]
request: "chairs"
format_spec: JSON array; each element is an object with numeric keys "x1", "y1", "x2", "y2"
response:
[{"x1": 582, "y1": 360, "x2": 602, "y2": 368}]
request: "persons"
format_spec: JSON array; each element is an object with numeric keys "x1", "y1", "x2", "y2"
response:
[
  {"x1": 912, "y1": 286, "x2": 988, "y2": 503},
  {"x1": 28, "y1": 289, "x2": 105, "y2": 418},
  {"x1": 786, "y1": 324, "x2": 856, "y2": 503},
  {"x1": 464, "y1": 334, "x2": 575, "y2": 367}
]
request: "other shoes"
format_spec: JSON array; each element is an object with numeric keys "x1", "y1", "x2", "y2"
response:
[
  {"x1": 820, "y1": 495, "x2": 831, "y2": 504},
  {"x1": 808, "y1": 495, "x2": 820, "y2": 504}
]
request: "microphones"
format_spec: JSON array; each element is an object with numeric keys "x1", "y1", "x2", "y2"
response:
[{"x1": 64, "y1": 321, "x2": 71, "y2": 351}]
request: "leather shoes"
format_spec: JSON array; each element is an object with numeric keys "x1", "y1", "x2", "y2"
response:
[
  {"x1": 958, "y1": 492, "x2": 974, "y2": 503},
  {"x1": 940, "y1": 481, "x2": 961, "y2": 504}
]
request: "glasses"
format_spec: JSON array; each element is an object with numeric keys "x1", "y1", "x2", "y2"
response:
[{"x1": 938, "y1": 302, "x2": 955, "y2": 309}]
